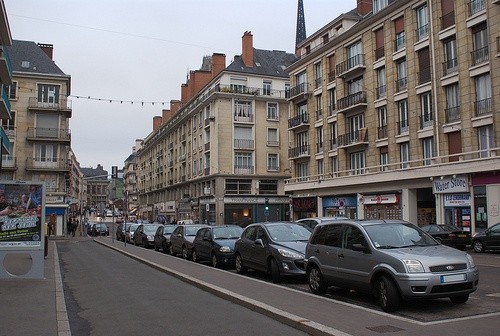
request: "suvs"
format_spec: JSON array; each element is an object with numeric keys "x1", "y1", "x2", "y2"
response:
[{"x1": 304, "y1": 219, "x2": 480, "y2": 310}]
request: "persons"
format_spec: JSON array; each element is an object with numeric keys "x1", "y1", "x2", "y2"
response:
[
  {"x1": 67, "y1": 218, "x2": 79, "y2": 237},
  {"x1": 0, "y1": 185, "x2": 42, "y2": 218}
]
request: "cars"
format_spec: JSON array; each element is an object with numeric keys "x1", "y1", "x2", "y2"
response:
[
  {"x1": 133, "y1": 224, "x2": 159, "y2": 249},
  {"x1": 115, "y1": 222, "x2": 136, "y2": 242},
  {"x1": 192, "y1": 225, "x2": 244, "y2": 269},
  {"x1": 233, "y1": 222, "x2": 313, "y2": 282},
  {"x1": 170, "y1": 224, "x2": 209, "y2": 260},
  {"x1": 471, "y1": 224, "x2": 500, "y2": 253},
  {"x1": 126, "y1": 224, "x2": 141, "y2": 245},
  {"x1": 85, "y1": 221, "x2": 97, "y2": 236},
  {"x1": 420, "y1": 224, "x2": 472, "y2": 251},
  {"x1": 153, "y1": 225, "x2": 176, "y2": 254},
  {"x1": 295, "y1": 217, "x2": 347, "y2": 233},
  {"x1": 93, "y1": 223, "x2": 110, "y2": 237}
]
